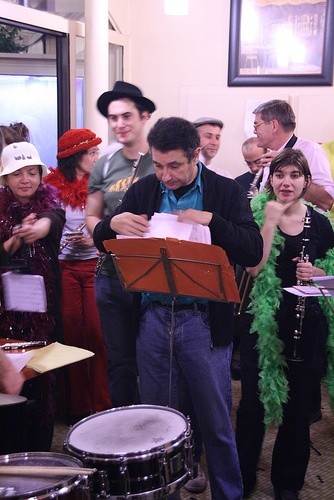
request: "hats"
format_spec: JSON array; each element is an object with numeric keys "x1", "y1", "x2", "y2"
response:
[
  {"x1": 97, "y1": 81, "x2": 156, "y2": 118},
  {"x1": 56, "y1": 129, "x2": 101, "y2": 160},
  {"x1": 0, "y1": 142, "x2": 47, "y2": 186},
  {"x1": 194, "y1": 117, "x2": 224, "y2": 128}
]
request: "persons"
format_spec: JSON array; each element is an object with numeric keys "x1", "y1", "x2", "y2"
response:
[
  {"x1": 247, "y1": 99, "x2": 334, "y2": 426},
  {"x1": 0, "y1": 138, "x2": 66, "y2": 456},
  {"x1": 90, "y1": 116, "x2": 265, "y2": 500},
  {"x1": 192, "y1": 117, "x2": 227, "y2": 178},
  {"x1": 235, "y1": 148, "x2": 334, "y2": 500},
  {"x1": 41, "y1": 126, "x2": 111, "y2": 425},
  {"x1": 83, "y1": 80, "x2": 209, "y2": 494},
  {"x1": 230, "y1": 135, "x2": 270, "y2": 383}
]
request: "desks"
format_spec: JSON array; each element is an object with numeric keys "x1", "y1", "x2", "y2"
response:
[{"x1": 0, "y1": 337, "x2": 95, "y2": 456}]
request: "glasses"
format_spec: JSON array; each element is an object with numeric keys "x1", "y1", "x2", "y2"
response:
[{"x1": 253, "y1": 123, "x2": 264, "y2": 131}]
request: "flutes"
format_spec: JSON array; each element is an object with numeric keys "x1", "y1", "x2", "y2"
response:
[
  {"x1": 92, "y1": 150, "x2": 146, "y2": 278},
  {"x1": 58, "y1": 222, "x2": 87, "y2": 254},
  {"x1": 286, "y1": 208, "x2": 313, "y2": 363}
]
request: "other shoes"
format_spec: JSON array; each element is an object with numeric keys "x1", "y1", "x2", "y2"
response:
[
  {"x1": 184, "y1": 468, "x2": 206, "y2": 491},
  {"x1": 274, "y1": 487, "x2": 298, "y2": 500}
]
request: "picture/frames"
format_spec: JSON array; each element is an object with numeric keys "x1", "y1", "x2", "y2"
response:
[{"x1": 228, "y1": 0, "x2": 334, "y2": 87}]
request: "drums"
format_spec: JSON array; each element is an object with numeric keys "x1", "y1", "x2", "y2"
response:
[
  {"x1": 0, "y1": 450, "x2": 92, "y2": 500},
  {"x1": 63, "y1": 404, "x2": 200, "y2": 500}
]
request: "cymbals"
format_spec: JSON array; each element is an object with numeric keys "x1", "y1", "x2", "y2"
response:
[{"x1": 0, "y1": 393, "x2": 28, "y2": 406}]
